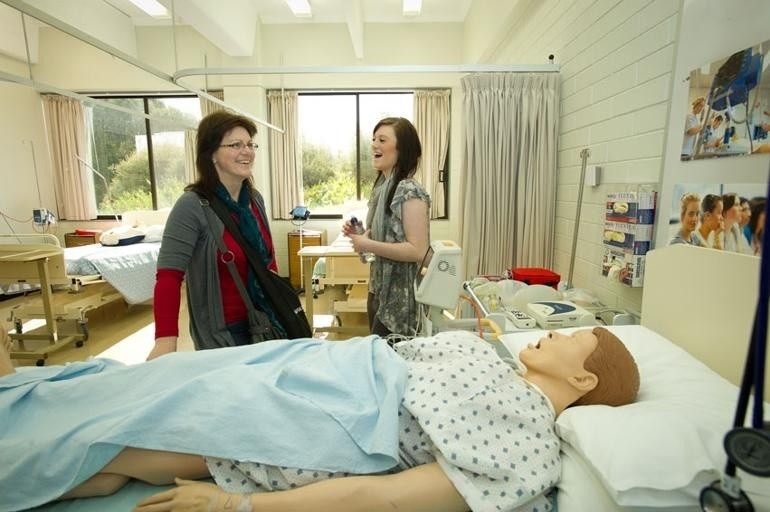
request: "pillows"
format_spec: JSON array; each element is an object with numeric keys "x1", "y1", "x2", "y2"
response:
[{"x1": 509, "y1": 327, "x2": 770, "y2": 511}]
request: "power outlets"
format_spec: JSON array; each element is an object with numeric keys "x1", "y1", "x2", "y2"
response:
[{"x1": 588, "y1": 165, "x2": 601, "y2": 187}]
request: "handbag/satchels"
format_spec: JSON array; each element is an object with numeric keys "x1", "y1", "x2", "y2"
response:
[
  {"x1": 260, "y1": 274, "x2": 315, "y2": 338},
  {"x1": 246, "y1": 308, "x2": 290, "y2": 343}
]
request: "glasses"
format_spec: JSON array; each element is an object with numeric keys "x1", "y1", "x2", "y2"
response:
[{"x1": 218, "y1": 140, "x2": 259, "y2": 152}]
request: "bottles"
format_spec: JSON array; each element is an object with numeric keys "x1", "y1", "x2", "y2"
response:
[{"x1": 350, "y1": 216, "x2": 377, "y2": 263}]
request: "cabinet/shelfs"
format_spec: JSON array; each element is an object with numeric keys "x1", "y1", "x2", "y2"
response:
[{"x1": 288, "y1": 228, "x2": 327, "y2": 292}]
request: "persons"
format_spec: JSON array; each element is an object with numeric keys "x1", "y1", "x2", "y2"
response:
[
  {"x1": 48, "y1": 325, "x2": 640, "y2": 512},
  {"x1": 341, "y1": 116, "x2": 433, "y2": 352},
  {"x1": 703, "y1": 115, "x2": 726, "y2": 152},
  {"x1": 145, "y1": 108, "x2": 289, "y2": 361},
  {"x1": 668, "y1": 193, "x2": 768, "y2": 259},
  {"x1": 683, "y1": 95, "x2": 706, "y2": 158}
]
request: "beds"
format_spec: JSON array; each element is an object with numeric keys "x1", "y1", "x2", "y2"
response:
[
  {"x1": 1, "y1": 210, "x2": 176, "y2": 364},
  {"x1": 298, "y1": 201, "x2": 375, "y2": 339}
]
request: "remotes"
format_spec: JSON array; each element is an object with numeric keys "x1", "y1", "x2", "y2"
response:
[{"x1": 506, "y1": 309, "x2": 536, "y2": 328}]
